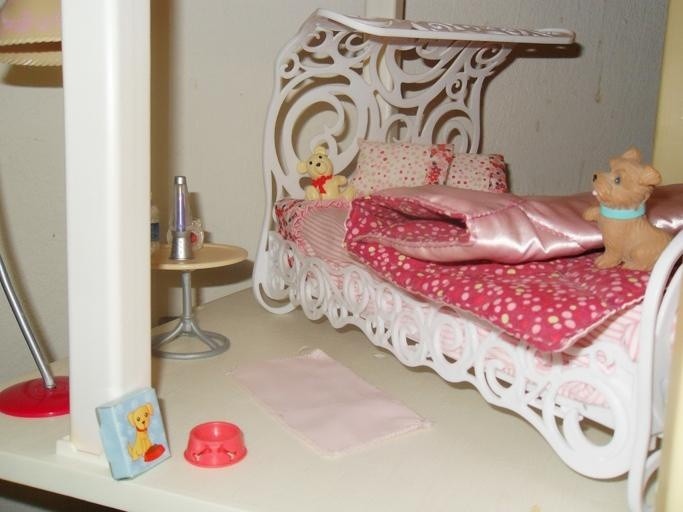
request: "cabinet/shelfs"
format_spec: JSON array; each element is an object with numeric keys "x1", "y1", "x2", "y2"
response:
[{"x1": 150, "y1": 196, "x2": 161, "y2": 256}]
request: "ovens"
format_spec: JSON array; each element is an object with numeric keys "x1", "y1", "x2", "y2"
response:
[{"x1": 252, "y1": 8, "x2": 683, "y2": 512}]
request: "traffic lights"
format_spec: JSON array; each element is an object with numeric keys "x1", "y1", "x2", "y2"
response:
[
  {"x1": 441, "y1": 151, "x2": 510, "y2": 196},
  {"x1": 354, "y1": 138, "x2": 455, "y2": 202}
]
